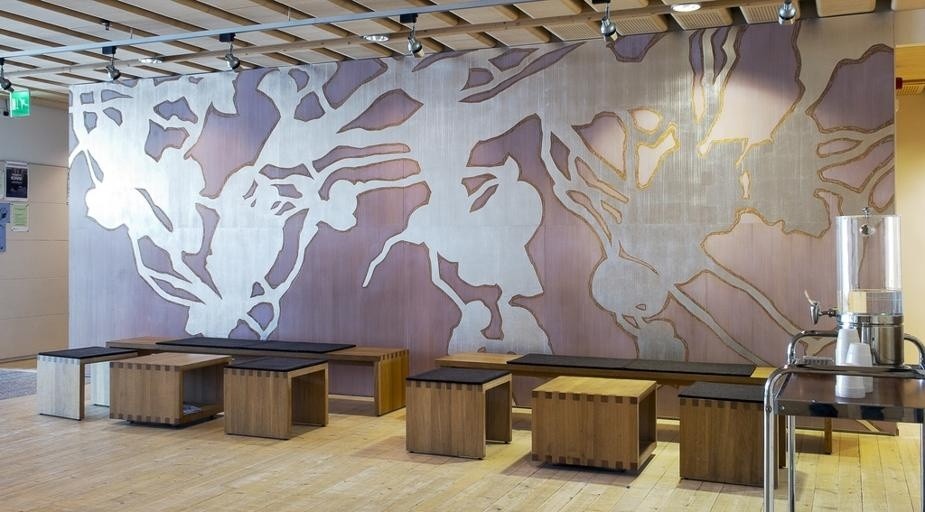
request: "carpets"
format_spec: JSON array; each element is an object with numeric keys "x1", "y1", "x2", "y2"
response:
[{"x1": 0, "y1": 367, "x2": 90, "y2": 400}]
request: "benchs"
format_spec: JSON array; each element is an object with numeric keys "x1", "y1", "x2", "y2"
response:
[
  {"x1": 106, "y1": 336, "x2": 410, "y2": 416},
  {"x1": 435, "y1": 351, "x2": 832, "y2": 456}
]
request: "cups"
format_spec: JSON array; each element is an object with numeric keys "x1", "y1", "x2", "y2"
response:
[{"x1": 834, "y1": 329, "x2": 877, "y2": 398}]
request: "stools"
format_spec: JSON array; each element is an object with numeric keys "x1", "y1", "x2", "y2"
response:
[
  {"x1": 531, "y1": 375, "x2": 658, "y2": 472},
  {"x1": 109, "y1": 352, "x2": 235, "y2": 428},
  {"x1": 36, "y1": 346, "x2": 137, "y2": 420},
  {"x1": 224, "y1": 355, "x2": 329, "y2": 440},
  {"x1": 405, "y1": 366, "x2": 513, "y2": 459},
  {"x1": 677, "y1": 381, "x2": 785, "y2": 489}
]
